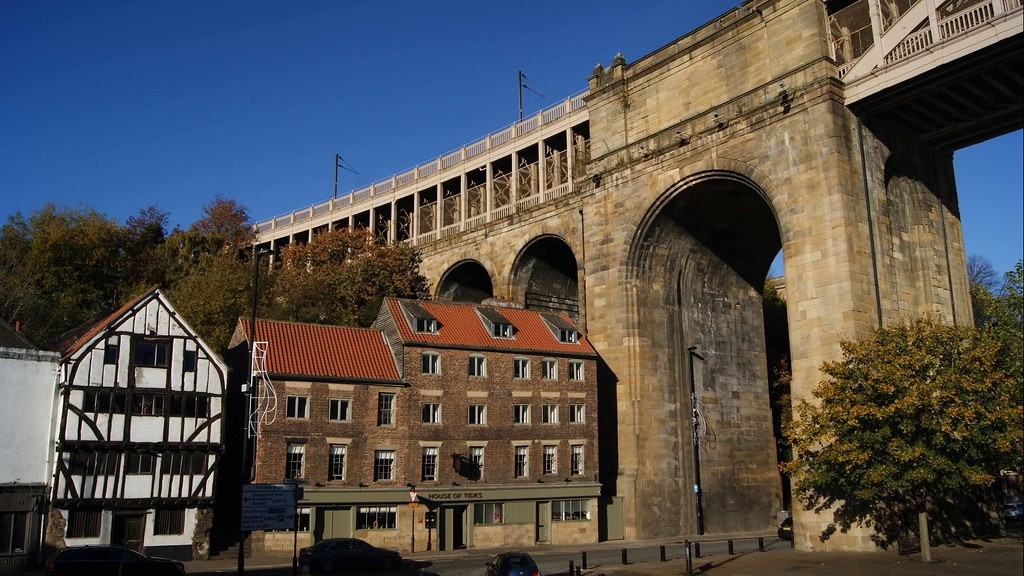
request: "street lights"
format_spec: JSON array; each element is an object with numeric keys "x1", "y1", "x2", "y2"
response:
[
  {"x1": 687, "y1": 345, "x2": 704, "y2": 535},
  {"x1": 237, "y1": 249, "x2": 277, "y2": 576}
]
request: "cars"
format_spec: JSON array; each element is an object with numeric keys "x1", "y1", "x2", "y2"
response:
[
  {"x1": 298, "y1": 538, "x2": 402, "y2": 575},
  {"x1": 485, "y1": 551, "x2": 542, "y2": 576}
]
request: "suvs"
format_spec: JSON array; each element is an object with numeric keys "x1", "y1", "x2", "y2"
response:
[{"x1": 44, "y1": 545, "x2": 186, "y2": 576}]
